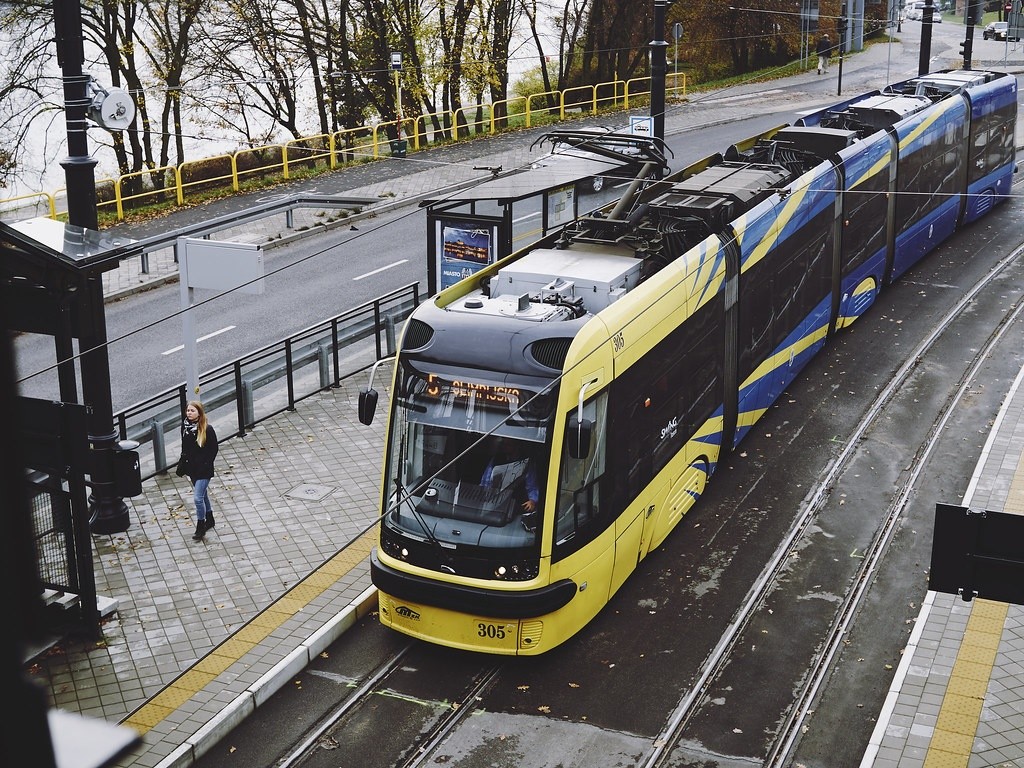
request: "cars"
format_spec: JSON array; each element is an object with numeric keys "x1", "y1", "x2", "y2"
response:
[
  {"x1": 983, "y1": 21, "x2": 1021, "y2": 41},
  {"x1": 918, "y1": 12, "x2": 942, "y2": 23},
  {"x1": 905, "y1": 8, "x2": 910, "y2": 18}
]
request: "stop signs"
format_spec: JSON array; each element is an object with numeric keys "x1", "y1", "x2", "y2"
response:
[{"x1": 1004, "y1": 2, "x2": 1012, "y2": 13}]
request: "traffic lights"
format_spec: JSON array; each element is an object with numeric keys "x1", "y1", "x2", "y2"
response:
[
  {"x1": 840, "y1": 16, "x2": 848, "y2": 33},
  {"x1": 959, "y1": 41, "x2": 966, "y2": 58}
]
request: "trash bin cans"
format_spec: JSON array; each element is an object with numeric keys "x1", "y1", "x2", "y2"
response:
[{"x1": 115, "y1": 440, "x2": 143, "y2": 498}]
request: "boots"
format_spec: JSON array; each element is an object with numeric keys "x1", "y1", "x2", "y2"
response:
[
  {"x1": 203, "y1": 510, "x2": 215, "y2": 533},
  {"x1": 192, "y1": 519, "x2": 206, "y2": 540}
]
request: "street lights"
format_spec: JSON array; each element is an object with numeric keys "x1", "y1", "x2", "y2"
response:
[{"x1": 886, "y1": 4, "x2": 906, "y2": 85}]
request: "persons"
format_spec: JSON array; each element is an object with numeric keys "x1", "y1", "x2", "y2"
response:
[
  {"x1": 478, "y1": 441, "x2": 538, "y2": 512},
  {"x1": 816, "y1": 34, "x2": 831, "y2": 75},
  {"x1": 176, "y1": 402, "x2": 219, "y2": 539}
]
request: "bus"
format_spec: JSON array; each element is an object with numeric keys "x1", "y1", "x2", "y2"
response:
[
  {"x1": 359, "y1": 68, "x2": 1017, "y2": 656},
  {"x1": 910, "y1": 3, "x2": 925, "y2": 19}
]
